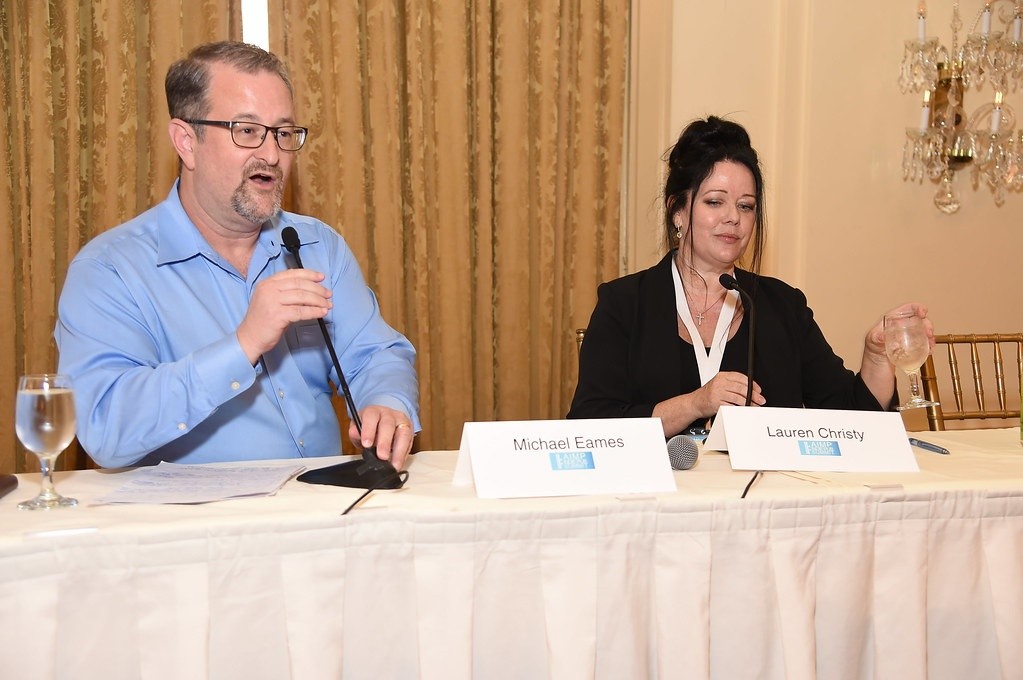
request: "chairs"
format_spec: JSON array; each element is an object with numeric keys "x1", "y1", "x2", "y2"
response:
[{"x1": 919, "y1": 332, "x2": 1023, "y2": 430}]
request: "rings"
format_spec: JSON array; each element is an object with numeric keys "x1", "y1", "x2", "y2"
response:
[{"x1": 395, "y1": 423, "x2": 411, "y2": 432}]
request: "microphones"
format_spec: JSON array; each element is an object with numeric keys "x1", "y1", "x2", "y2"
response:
[
  {"x1": 281, "y1": 226, "x2": 402, "y2": 490},
  {"x1": 666, "y1": 435, "x2": 698, "y2": 471},
  {"x1": 719, "y1": 272, "x2": 755, "y2": 406}
]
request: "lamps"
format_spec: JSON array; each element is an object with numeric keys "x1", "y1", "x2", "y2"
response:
[{"x1": 897, "y1": 0, "x2": 1023, "y2": 214}]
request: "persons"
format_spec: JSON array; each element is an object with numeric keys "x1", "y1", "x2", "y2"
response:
[
  {"x1": 53, "y1": 40, "x2": 423, "y2": 473},
  {"x1": 565, "y1": 115, "x2": 933, "y2": 442}
]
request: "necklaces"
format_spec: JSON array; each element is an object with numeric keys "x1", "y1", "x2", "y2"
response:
[{"x1": 682, "y1": 281, "x2": 728, "y2": 326}]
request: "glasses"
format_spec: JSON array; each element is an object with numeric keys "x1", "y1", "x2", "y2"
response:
[{"x1": 179, "y1": 117, "x2": 309, "y2": 151}]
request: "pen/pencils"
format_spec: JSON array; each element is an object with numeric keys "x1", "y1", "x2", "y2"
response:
[{"x1": 909, "y1": 438, "x2": 950, "y2": 455}]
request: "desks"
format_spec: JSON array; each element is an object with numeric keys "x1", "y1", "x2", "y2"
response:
[{"x1": 0, "y1": 436, "x2": 1023, "y2": 680}]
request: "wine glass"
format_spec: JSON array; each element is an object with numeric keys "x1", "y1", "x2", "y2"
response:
[
  {"x1": 884, "y1": 313, "x2": 940, "y2": 411},
  {"x1": 15, "y1": 374, "x2": 78, "y2": 509}
]
